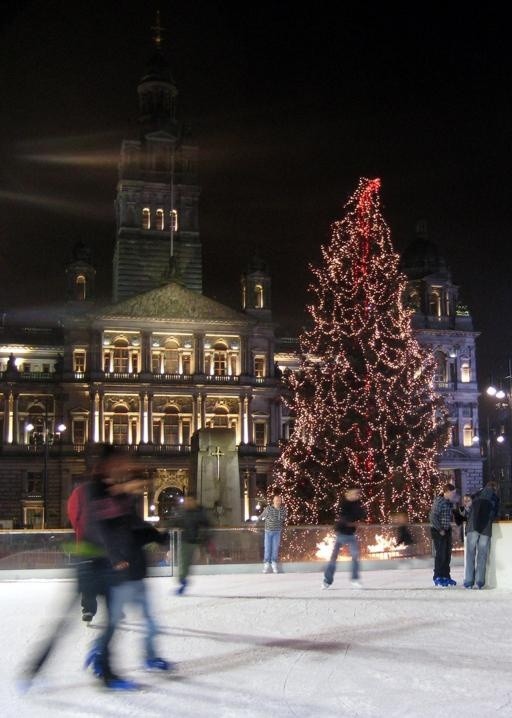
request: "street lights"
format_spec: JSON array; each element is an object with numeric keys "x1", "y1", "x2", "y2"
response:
[
  {"x1": 486, "y1": 357, "x2": 512, "y2": 399},
  {"x1": 485, "y1": 408, "x2": 509, "y2": 488},
  {"x1": 22, "y1": 397, "x2": 66, "y2": 530}
]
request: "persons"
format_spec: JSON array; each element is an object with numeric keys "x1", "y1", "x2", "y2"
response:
[
  {"x1": 426, "y1": 482, "x2": 456, "y2": 587},
  {"x1": 69, "y1": 443, "x2": 173, "y2": 690},
  {"x1": 461, "y1": 479, "x2": 499, "y2": 589},
  {"x1": 66, "y1": 471, "x2": 130, "y2": 625},
  {"x1": 251, "y1": 494, "x2": 287, "y2": 573},
  {"x1": 319, "y1": 487, "x2": 365, "y2": 589},
  {"x1": 161, "y1": 491, "x2": 213, "y2": 595},
  {"x1": 452, "y1": 492, "x2": 471, "y2": 528}
]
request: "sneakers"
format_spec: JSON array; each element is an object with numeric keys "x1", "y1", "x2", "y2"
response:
[
  {"x1": 263, "y1": 562, "x2": 279, "y2": 570},
  {"x1": 81, "y1": 607, "x2": 94, "y2": 622}
]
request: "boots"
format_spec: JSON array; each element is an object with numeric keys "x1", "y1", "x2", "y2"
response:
[
  {"x1": 445, "y1": 578, "x2": 457, "y2": 586},
  {"x1": 434, "y1": 577, "x2": 449, "y2": 587},
  {"x1": 463, "y1": 581, "x2": 483, "y2": 590}
]
